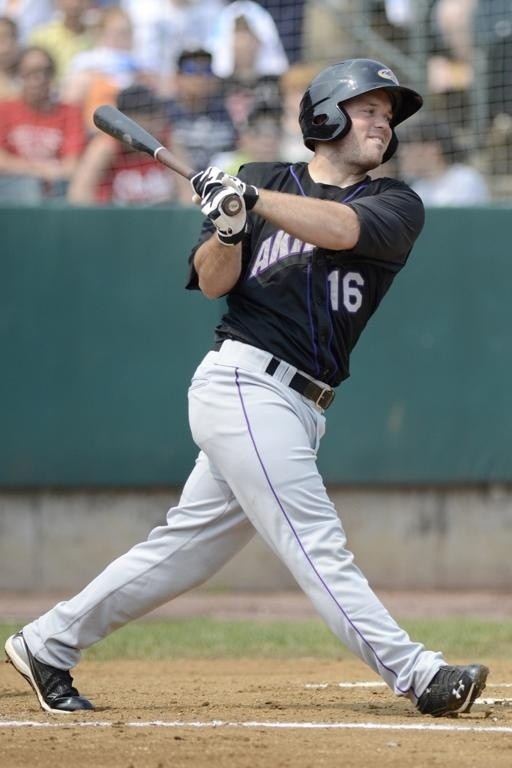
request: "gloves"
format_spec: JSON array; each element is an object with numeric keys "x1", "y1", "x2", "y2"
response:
[{"x1": 190, "y1": 167, "x2": 259, "y2": 247}]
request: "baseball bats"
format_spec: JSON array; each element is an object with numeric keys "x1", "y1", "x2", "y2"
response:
[{"x1": 94, "y1": 104, "x2": 241, "y2": 216}]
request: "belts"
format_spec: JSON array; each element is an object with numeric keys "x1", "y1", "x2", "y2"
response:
[{"x1": 211, "y1": 343, "x2": 336, "y2": 414}]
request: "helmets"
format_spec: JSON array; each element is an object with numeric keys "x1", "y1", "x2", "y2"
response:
[{"x1": 298, "y1": 59, "x2": 423, "y2": 164}]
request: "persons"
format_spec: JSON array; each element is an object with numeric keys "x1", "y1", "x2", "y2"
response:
[
  {"x1": 0, "y1": 0, "x2": 318, "y2": 209},
  {"x1": 3, "y1": 58, "x2": 489, "y2": 715},
  {"x1": 393, "y1": 114, "x2": 489, "y2": 209},
  {"x1": 430, "y1": 0, "x2": 512, "y2": 127}
]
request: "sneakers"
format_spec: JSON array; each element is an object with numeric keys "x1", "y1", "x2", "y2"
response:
[
  {"x1": 4, "y1": 628, "x2": 94, "y2": 714},
  {"x1": 415, "y1": 664, "x2": 488, "y2": 717}
]
